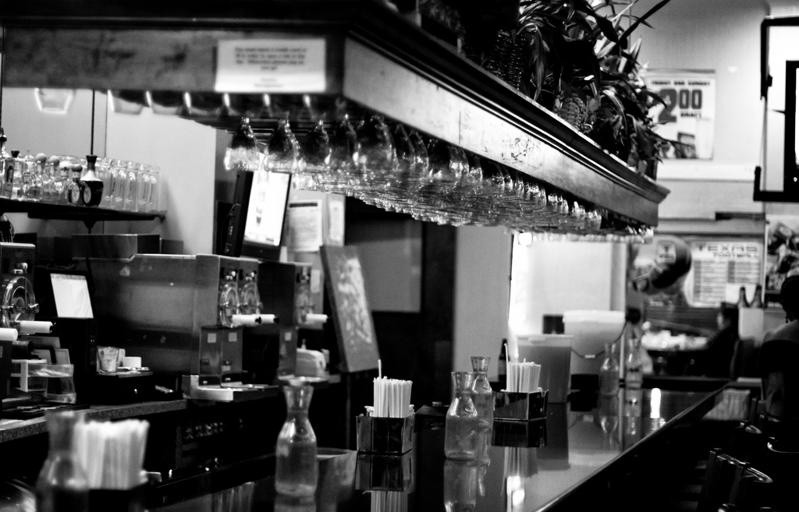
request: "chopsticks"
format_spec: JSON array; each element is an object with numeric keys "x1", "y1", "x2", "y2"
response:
[
  {"x1": 505, "y1": 344, "x2": 541, "y2": 393},
  {"x1": 73, "y1": 417, "x2": 147, "y2": 494},
  {"x1": 500, "y1": 446, "x2": 537, "y2": 495},
  {"x1": 371, "y1": 488, "x2": 409, "y2": 512},
  {"x1": 372, "y1": 360, "x2": 413, "y2": 419}
]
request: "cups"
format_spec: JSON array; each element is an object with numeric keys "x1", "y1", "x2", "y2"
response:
[
  {"x1": 186, "y1": 94, "x2": 225, "y2": 123},
  {"x1": 117, "y1": 348, "x2": 126, "y2": 370},
  {"x1": 225, "y1": 94, "x2": 269, "y2": 118},
  {"x1": 264, "y1": 95, "x2": 311, "y2": 116},
  {"x1": 225, "y1": 108, "x2": 258, "y2": 173},
  {"x1": 37, "y1": 90, "x2": 74, "y2": 116},
  {"x1": 99, "y1": 348, "x2": 118, "y2": 372},
  {"x1": 147, "y1": 93, "x2": 185, "y2": 117},
  {"x1": 123, "y1": 356, "x2": 142, "y2": 368},
  {"x1": 110, "y1": 92, "x2": 145, "y2": 114}
]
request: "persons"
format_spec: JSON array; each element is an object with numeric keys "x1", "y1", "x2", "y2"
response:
[
  {"x1": 769, "y1": 254, "x2": 799, "y2": 290},
  {"x1": 738, "y1": 275, "x2": 798, "y2": 453}
]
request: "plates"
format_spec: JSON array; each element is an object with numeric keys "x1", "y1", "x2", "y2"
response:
[{"x1": 118, "y1": 367, "x2": 149, "y2": 371}]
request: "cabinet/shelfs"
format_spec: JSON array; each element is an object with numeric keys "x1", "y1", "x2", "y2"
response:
[{"x1": 5, "y1": 10, "x2": 671, "y2": 236}]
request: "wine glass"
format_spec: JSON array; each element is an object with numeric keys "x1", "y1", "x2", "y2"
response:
[{"x1": 244, "y1": 105, "x2": 657, "y2": 246}]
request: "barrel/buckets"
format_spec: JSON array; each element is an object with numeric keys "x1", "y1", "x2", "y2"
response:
[{"x1": 563, "y1": 310, "x2": 627, "y2": 392}]
